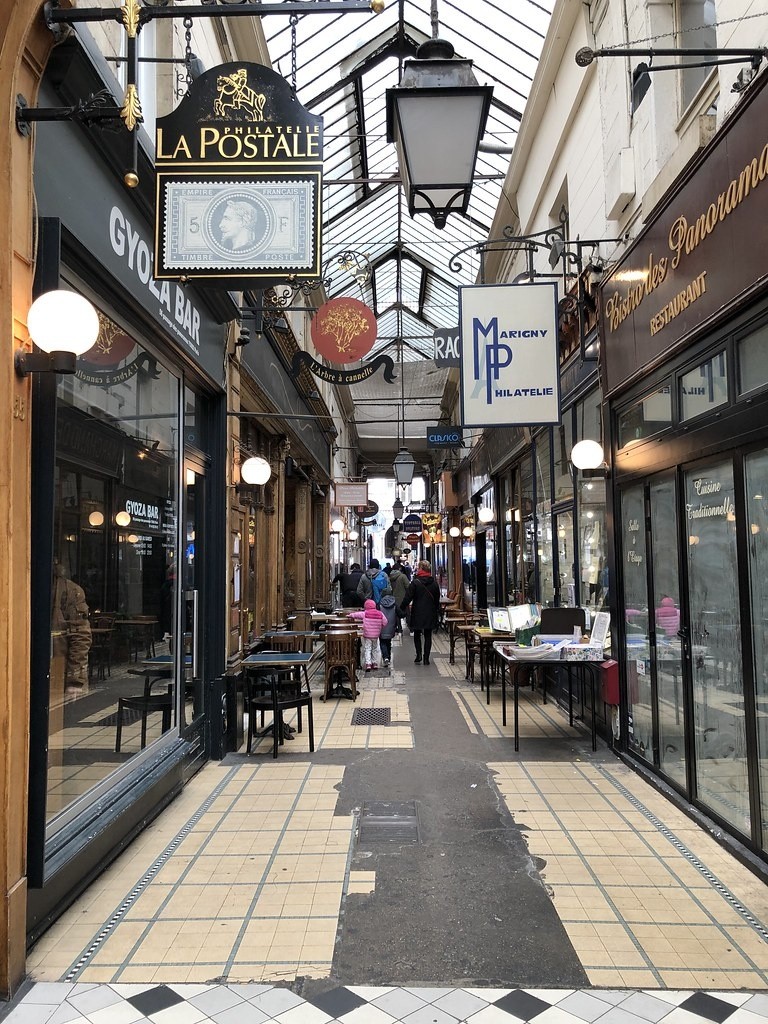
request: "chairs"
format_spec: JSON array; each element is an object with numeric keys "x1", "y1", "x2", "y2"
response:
[
  {"x1": 442, "y1": 591, "x2": 488, "y2": 679},
  {"x1": 271, "y1": 613, "x2": 358, "y2": 703},
  {"x1": 132, "y1": 615, "x2": 157, "y2": 658},
  {"x1": 89, "y1": 616, "x2": 115, "y2": 679},
  {"x1": 243, "y1": 668, "x2": 314, "y2": 759},
  {"x1": 115, "y1": 669, "x2": 173, "y2": 752}
]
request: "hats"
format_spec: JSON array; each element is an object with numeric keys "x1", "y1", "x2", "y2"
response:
[
  {"x1": 381, "y1": 588, "x2": 392, "y2": 596},
  {"x1": 370, "y1": 559, "x2": 379, "y2": 569}
]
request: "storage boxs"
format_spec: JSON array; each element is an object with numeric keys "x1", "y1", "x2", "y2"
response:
[{"x1": 560, "y1": 644, "x2": 603, "y2": 661}]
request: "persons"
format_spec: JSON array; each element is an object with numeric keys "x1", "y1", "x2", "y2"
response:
[
  {"x1": 625, "y1": 597, "x2": 680, "y2": 642},
  {"x1": 526, "y1": 562, "x2": 537, "y2": 605},
  {"x1": 382, "y1": 560, "x2": 413, "y2": 583},
  {"x1": 399, "y1": 560, "x2": 440, "y2": 665},
  {"x1": 342, "y1": 563, "x2": 364, "y2": 608},
  {"x1": 331, "y1": 567, "x2": 350, "y2": 603},
  {"x1": 388, "y1": 563, "x2": 410, "y2": 634},
  {"x1": 357, "y1": 558, "x2": 393, "y2": 611},
  {"x1": 376, "y1": 587, "x2": 407, "y2": 667},
  {"x1": 46, "y1": 554, "x2": 92, "y2": 812},
  {"x1": 461, "y1": 557, "x2": 478, "y2": 595},
  {"x1": 346, "y1": 599, "x2": 388, "y2": 672}
]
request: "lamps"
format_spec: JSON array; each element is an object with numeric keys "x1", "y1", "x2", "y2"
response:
[
  {"x1": 112, "y1": 511, "x2": 131, "y2": 530},
  {"x1": 479, "y1": 508, "x2": 495, "y2": 526},
  {"x1": 632, "y1": 61, "x2": 652, "y2": 115},
  {"x1": 392, "y1": 486, "x2": 405, "y2": 519},
  {"x1": 235, "y1": 457, "x2": 271, "y2": 495},
  {"x1": 392, "y1": 518, "x2": 401, "y2": 533},
  {"x1": 329, "y1": 519, "x2": 344, "y2": 535},
  {"x1": 386, "y1": 0, "x2": 494, "y2": 230},
  {"x1": 88, "y1": 511, "x2": 104, "y2": 530},
  {"x1": 571, "y1": 440, "x2": 610, "y2": 481},
  {"x1": 393, "y1": 246, "x2": 416, "y2": 490},
  {"x1": 14, "y1": 289, "x2": 99, "y2": 381},
  {"x1": 506, "y1": 509, "x2": 520, "y2": 525},
  {"x1": 348, "y1": 531, "x2": 358, "y2": 542},
  {"x1": 128, "y1": 535, "x2": 138, "y2": 548},
  {"x1": 449, "y1": 526, "x2": 460, "y2": 539},
  {"x1": 463, "y1": 527, "x2": 472, "y2": 543}
]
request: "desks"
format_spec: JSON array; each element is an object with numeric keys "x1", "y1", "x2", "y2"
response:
[
  {"x1": 472, "y1": 629, "x2": 515, "y2": 706},
  {"x1": 141, "y1": 655, "x2": 191, "y2": 750},
  {"x1": 433, "y1": 598, "x2": 454, "y2": 634},
  {"x1": 241, "y1": 653, "x2": 314, "y2": 741},
  {"x1": 444, "y1": 617, "x2": 488, "y2": 665},
  {"x1": 319, "y1": 624, "x2": 363, "y2": 682},
  {"x1": 259, "y1": 631, "x2": 314, "y2": 681},
  {"x1": 494, "y1": 646, "x2": 606, "y2": 752},
  {"x1": 311, "y1": 630, "x2": 363, "y2": 701},
  {"x1": 91, "y1": 629, "x2": 115, "y2": 678},
  {"x1": 286, "y1": 607, "x2": 364, "y2": 631},
  {"x1": 636, "y1": 655, "x2": 707, "y2": 730},
  {"x1": 95, "y1": 619, "x2": 158, "y2": 663},
  {"x1": 457, "y1": 625, "x2": 480, "y2": 683}
]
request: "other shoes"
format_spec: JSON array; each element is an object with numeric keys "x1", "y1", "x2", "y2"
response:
[
  {"x1": 383, "y1": 660, "x2": 388, "y2": 667},
  {"x1": 372, "y1": 663, "x2": 377, "y2": 668},
  {"x1": 364, "y1": 664, "x2": 371, "y2": 670},
  {"x1": 414, "y1": 653, "x2": 422, "y2": 662},
  {"x1": 424, "y1": 655, "x2": 430, "y2": 665}
]
